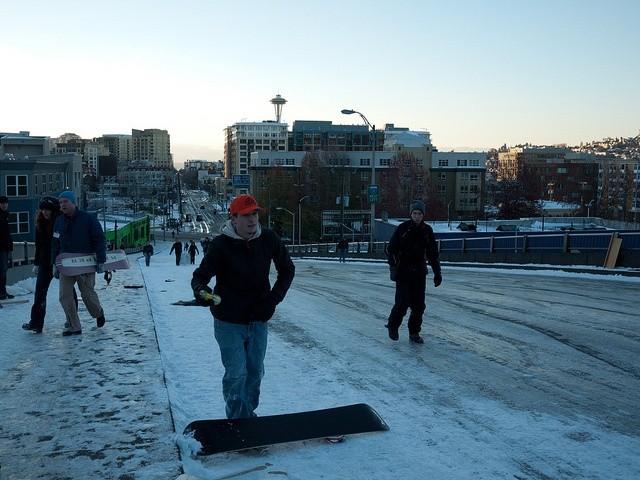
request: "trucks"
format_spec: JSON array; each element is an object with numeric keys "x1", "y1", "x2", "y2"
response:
[{"x1": 197, "y1": 214, "x2": 202, "y2": 221}]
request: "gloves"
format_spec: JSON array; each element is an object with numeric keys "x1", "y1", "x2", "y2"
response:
[
  {"x1": 53, "y1": 264, "x2": 59, "y2": 279},
  {"x1": 196, "y1": 285, "x2": 215, "y2": 307},
  {"x1": 389, "y1": 266, "x2": 397, "y2": 280},
  {"x1": 31, "y1": 265, "x2": 39, "y2": 274},
  {"x1": 434, "y1": 274, "x2": 442, "y2": 287},
  {"x1": 96, "y1": 262, "x2": 103, "y2": 274}
]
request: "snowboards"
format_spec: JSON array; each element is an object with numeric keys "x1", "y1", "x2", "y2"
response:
[
  {"x1": 182, "y1": 404, "x2": 391, "y2": 457},
  {"x1": 55, "y1": 249, "x2": 130, "y2": 277}
]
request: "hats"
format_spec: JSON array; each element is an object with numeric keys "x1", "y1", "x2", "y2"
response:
[
  {"x1": 0, "y1": 196, "x2": 9, "y2": 203},
  {"x1": 39, "y1": 195, "x2": 60, "y2": 212},
  {"x1": 409, "y1": 200, "x2": 426, "y2": 216},
  {"x1": 230, "y1": 195, "x2": 265, "y2": 216},
  {"x1": 58, "y1": 190, "x2": 78, "y2": 208}
]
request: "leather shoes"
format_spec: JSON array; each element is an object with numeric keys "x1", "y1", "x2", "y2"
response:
[
  {"x1": 22, "y1": 323, "x2": 42, "y2": 333},
  {"x1": 63, "y1": 329, "x2": 82, "y2": 336},
  {"x1": 96, "y1": 310, "x2": 105, "y2": 328}
]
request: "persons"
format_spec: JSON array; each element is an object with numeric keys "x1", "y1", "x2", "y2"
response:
[
  {"x1": 169, "y1": 237, "x2": 182, "y2": 266},
  {"x1": 50, "y1": 189, "x2": 108, "y2": 337},
  {"x1": 21, "y1": 195, "x2": 79, "y2": 334},
  {"x1": 188, "y1": 242, "x2": 200, "y2": 264},
  {"x1": 188, "y1": 240, "x2": 192, "y2": 248},
  {"x1": 142, "y1": 240, "x2": 155, "y2": 267},
  {"x1": 200, "y1": 236, "x2": 211, "y2": 256},
  {"x1": 336, "y1": 235, "x2": 350, "y2": 263},
  {"x1": 0, "y1": 195, "x2": 15, "y2": 301},
  {"x1": 171, "y1": 230, "x2": 176, "y2": 237},
  {"x1": 382, "y1": 199, "x2": 444, "y2": 345},
  {"x1": 185, "y1": 242, "x2": 188, "y2": 251},
  {"x1": 190, "y1": 193, "x2": 297, "y2": 420}
]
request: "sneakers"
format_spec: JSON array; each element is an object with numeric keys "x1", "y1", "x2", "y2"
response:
[
  {"x1": 388, "y1": 327, "x2": 399, "y2": 341},
  {"x1": 410, "y1": 333, "x2": 424, "y2": 344}
]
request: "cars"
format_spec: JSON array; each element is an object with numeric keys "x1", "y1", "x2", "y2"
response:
[{"x1": 201, "y1": 206, "x2": 204, "y2": 209}]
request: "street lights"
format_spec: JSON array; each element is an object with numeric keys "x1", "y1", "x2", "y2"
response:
[
  {"x1": 103, "y1": 160, "x2": 138, "y2": 235},
  {"x1": 276, "y1": 207, "x2": 295, "y2": 253},
  {"x1": 341, "y1": 109, "x2": 375, "y2": 252},
  {"x1": 152, "y1": 192, "x2": 165, "y2": 246},
  {"x1": 178, "y1": 169, "x2": 183, "y2": 214},
  {"x1": 299, "y1": 196, "x2": 309, "y2": 254}
]
